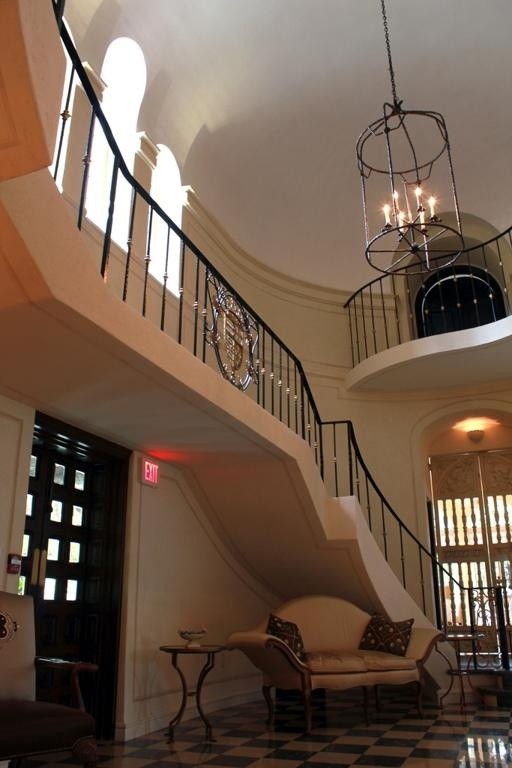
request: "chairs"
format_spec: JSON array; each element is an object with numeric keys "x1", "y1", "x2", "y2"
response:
[{"x1": 1, "y1": 591, "x2": 100, "y2": 768}]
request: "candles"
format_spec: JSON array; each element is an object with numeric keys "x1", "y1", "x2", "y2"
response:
[
  {"x1": 397, "y1": 212, "x2": 403, "y2": 232},
  {"x1": 428, "y1": 194, "x2": 436, "y2": 218},
  {"x1": 414, "y1": 187, "x2": 421, "y2": 207},
  {"x1": 382, "y1": 204, "x2": 392, "y2": 225},
  {"x1": 419, "y1": 212, "x2": 425, "y2": 229},
  {"x1": 393, "y1": 189, "x2": 399, "y2": 210}
]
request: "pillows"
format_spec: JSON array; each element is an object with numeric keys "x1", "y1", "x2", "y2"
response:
[
  {"x1": 357, "y1": 611, "x2": 414, "y2": 656},
  {"x1": 267, "y1": 614, "x2": 308, "y2": 661}
]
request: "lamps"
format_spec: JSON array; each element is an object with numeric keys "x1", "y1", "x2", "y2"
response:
[{"x1": 467, "y1": 429, "x2": 484, "y2": 442}]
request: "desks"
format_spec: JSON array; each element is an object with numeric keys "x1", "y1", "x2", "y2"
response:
[
  {"x1": 439, "y1": 631, "x2": 485, "y2": 707},
  {"x1": 158, "y1": 643, "x2": 228, "y2": 743}
]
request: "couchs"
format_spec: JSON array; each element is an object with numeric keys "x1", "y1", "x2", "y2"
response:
[{"x1": 227, "y1": 593, "x2": 447, "y2": 734}]
